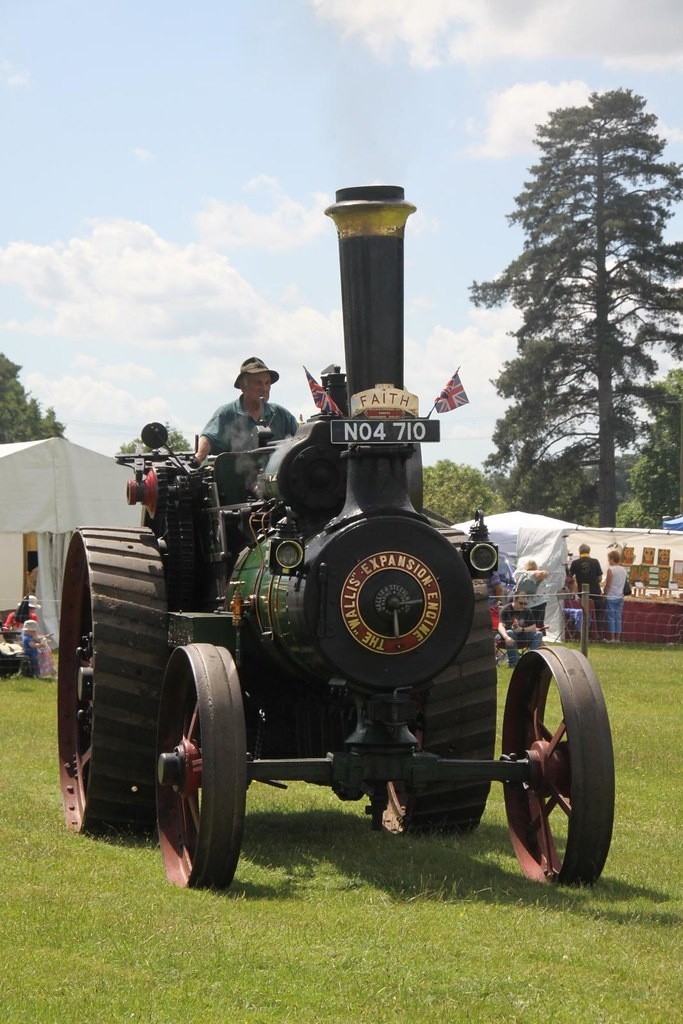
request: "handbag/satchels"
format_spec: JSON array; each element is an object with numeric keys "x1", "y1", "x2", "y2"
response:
[{"x1": 623, "y1": 576, "x2": 632, "y2": 596}]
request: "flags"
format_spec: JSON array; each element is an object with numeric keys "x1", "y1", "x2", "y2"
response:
[
  {"x1": 303, "y1": 367, "x2": 346, "y2": 417},
  {"x1": 435, "y1": 373, "x2": 470, "y2": 414}
]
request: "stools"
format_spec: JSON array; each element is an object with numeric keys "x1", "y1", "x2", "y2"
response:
[{"x1": 15, "y1": 652, "x2": 40, "y2": 679}]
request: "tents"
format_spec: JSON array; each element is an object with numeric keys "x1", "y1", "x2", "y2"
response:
[
  {"x1": 0, "y1": 436, "x2": 143, "y2": 651},
  {"x1": 451, "y1": 510, "x2": 683, "y2": 642}
]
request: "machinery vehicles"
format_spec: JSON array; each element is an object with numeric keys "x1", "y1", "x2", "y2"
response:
[{"x1": 57, "y1": 186, "x2": 615, "y2": 890}]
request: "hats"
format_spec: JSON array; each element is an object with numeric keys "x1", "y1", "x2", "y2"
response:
[
  {"x1": 18, "y1": 595, "x2": 42, "y2": 609},
  {"x1": 21, "y1": 619, "x2": 40, "y2": 631},
  {"x1": 234, "y1": 357, "x2": 279, "y2": 388}
]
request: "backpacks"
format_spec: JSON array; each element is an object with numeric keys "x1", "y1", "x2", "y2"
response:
[{"x1": 518, "y1": 571, "x2": 537, "y2": 594}]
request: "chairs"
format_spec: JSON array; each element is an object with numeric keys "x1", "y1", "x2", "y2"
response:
[
  {"x1": 488, "y1": 607, "x2": 534, "y2": 669},
  {"x1": 552, "y1": 592, "x2": 591, "y2": 644}
]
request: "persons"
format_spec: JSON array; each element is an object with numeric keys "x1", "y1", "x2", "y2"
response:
[
  {"x1": 602, "y1": 551, "x2": 627, "y2": 644},
  {"x1": 508, "y1": 560, "x2": 548, "y2": 605},
  {"x1": 486, "y1": 568, "x2": 504, "y2": 633},
  {"x1": 564, "y1": 544, "x2": 605, "y2": 643},
  {"x1": 3, "y1": 596, "x2": 42, "y2": 630},
  {"x1": 21, "y1": 620, "x2": 46, "y2": 680},
  {"x1": 188, "y1": 358, "x2": 299, "y2": 469},
  {"x1": 498, "y1": 590, "x2": 544, "y2": 670}
]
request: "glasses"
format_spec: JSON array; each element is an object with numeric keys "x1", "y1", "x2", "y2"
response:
[{"x1": 516, "y1": 600, "x2": 528, "y2": 606}]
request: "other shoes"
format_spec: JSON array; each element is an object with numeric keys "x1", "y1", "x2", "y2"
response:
[
  {"x1": 604, "y1": 639, "x2": 615, "y2": 645},
  {"x1": 615, "y1": 640, "x2": 620, "y2": 644}
]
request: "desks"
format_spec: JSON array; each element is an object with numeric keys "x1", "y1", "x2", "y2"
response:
[
  {"x1": 564, "y1": 594, "x2": 683, "y2": 642},
  {"x1": 0, "y1": 630, "x2": 23, "y2": 635}
]
